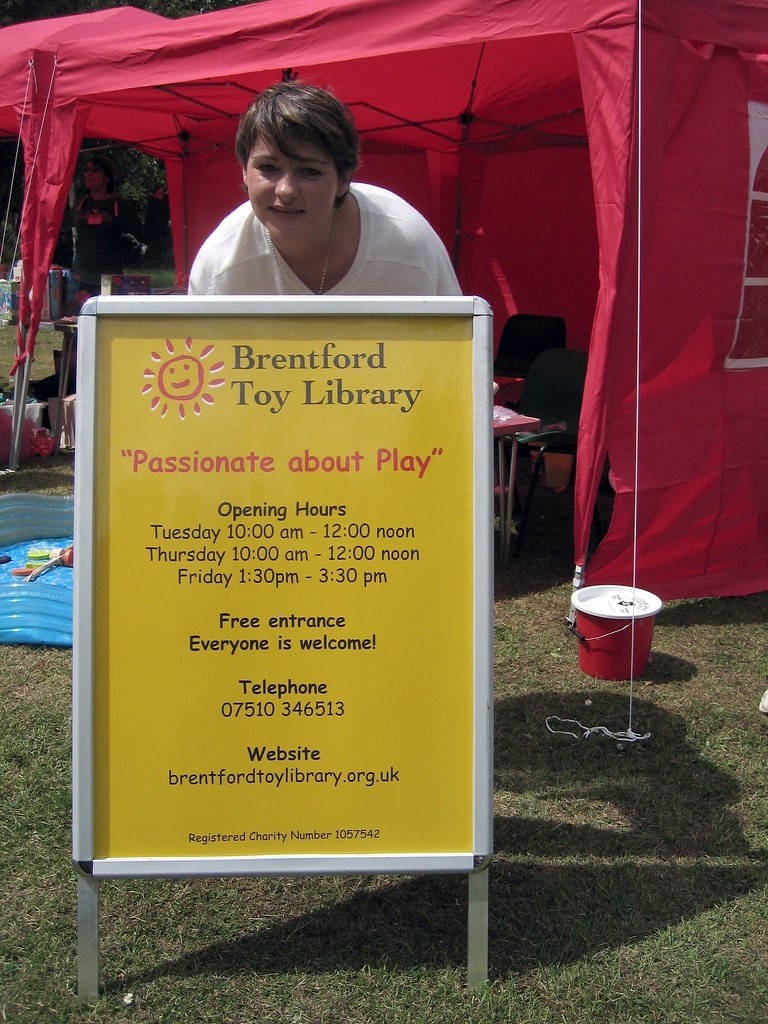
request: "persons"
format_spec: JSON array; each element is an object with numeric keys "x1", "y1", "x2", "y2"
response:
[
  {"x1": 186, "y1": 78, "x2": 464, "y2": 296},
  {"x1": 14, "y1": 152, "x2": 166, "y2": 396}
]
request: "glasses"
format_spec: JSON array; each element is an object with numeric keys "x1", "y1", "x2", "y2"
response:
[{"x1": 84, "y1": 167, "x2": 104, "y2": 173}]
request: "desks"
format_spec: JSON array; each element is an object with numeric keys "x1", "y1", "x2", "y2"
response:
[
  {"x1": 0, "y1": 319, "x2": 76, "y2": 454},
  {"x1": 494, "y1": 406, "x2": 541, "y2": 568}
]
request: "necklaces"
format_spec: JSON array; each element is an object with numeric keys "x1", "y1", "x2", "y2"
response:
[{"x1": 264, "y1": 207, "x2": 341, "y2": 295}]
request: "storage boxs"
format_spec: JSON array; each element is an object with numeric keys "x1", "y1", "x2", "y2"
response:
[
  {"x1": 47, "y1": 393, "x2": 76, "y2": 449},
  {"x1": 0, "y1": 267, "x2": 81, "y2": 324},
  {"x1": 0, "y1": 399, "x2": 48, "y2": 427},
  {"x1": 101, "y1": 275, "x2": 151, "y2": 297}
]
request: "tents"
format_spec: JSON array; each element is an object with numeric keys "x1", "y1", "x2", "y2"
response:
[{"x1": 0, "y1": 0, "x2": 768, "y2": 630}]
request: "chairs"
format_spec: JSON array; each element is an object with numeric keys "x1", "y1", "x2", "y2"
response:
[
  {"x1": 502, "y1": 348, "x2": 606, "y2": 557},
  {"x1": 493, "y1": 314, "x2": 569, "y2": 507}
]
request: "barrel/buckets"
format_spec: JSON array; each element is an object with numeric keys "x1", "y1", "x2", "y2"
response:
[
  {"x1": 568, "y1": 584, "x2": 664, "y2": 682},
  {"x1": 529, "y1": 451, "x2": 574, "y2": 488}
]
